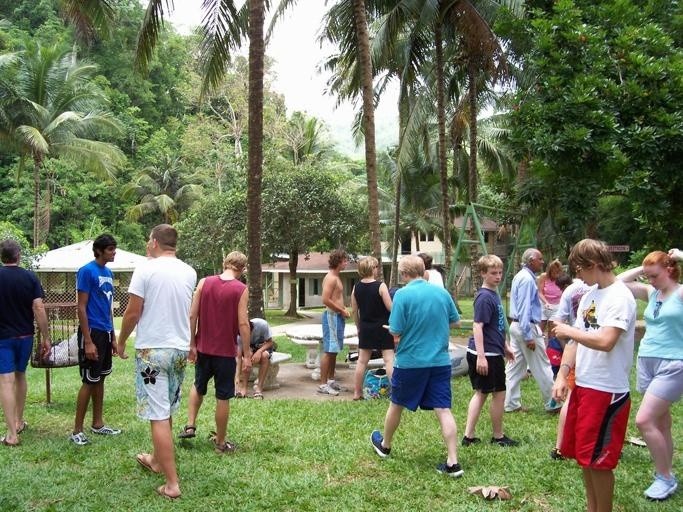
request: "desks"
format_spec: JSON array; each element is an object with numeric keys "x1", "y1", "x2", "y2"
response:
[{"x1": 286, "y1": 323, "x2": 361, "y2": 380}]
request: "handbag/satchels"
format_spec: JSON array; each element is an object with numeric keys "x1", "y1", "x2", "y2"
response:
[{"x1": 362, "y1": 370, "x2": 390, "y2": 401}]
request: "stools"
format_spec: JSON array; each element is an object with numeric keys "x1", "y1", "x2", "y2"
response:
[
  {"x1": 344, "y1": 338, "x2": 360, "y2": 372},
  {"x1": 294, "y1": 338, "x2": 321, "y2": 369},
  {"x1": 246, "y1": 351, "x2": 292, "y2": 391}
]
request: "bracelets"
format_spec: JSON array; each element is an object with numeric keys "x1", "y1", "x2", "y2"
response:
[{"x1": 638, "y1": 266, "x2": 644, "y2": 277}]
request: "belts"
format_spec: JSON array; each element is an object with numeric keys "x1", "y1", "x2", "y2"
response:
[{"x1": 511, "y1": 318, "x2": 534, "y2": 323}]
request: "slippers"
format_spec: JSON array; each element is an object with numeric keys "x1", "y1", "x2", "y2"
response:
[
  {"x1": 354, "y1": 396, "x2": 364, "y2": 400},
  {"x1": 0, "y1": 436, "x2": 19, "y2": 446},
  {"x1": 136, "y1": 453, "x2": 160, "y2": 474},
  {"x1": 17, "y1": 421, "x2": 27, "y2": 434},
  {"x1": 157, "y1": 484, "x2": 182, "y2": 499}
]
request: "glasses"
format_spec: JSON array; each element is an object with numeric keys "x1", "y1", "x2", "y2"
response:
[
  {"x1": 643, "y1": 272, "x2": 661, "y2": 280},
  {"x1": 575, "y1": 265, "x2": 584, "y2": 275}
]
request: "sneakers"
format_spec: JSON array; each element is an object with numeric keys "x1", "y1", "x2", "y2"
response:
[
  {"x1": 235, "y1": 392, "x2": 247, "y2": 398},
  {"x1": 547, "y1": 407, "x2": 561, "y2": 415},
  {"x1": 317, "y1": 384, "x2": 339, "y2": 396},
  {"x1": 329, "y1": 382, "x2": 348, "y2": 392},
  {"x1": 550, "y1": 448, "x2": 566, "y2": 460},
  {"x1": 505, "y1": 407, "x2": 528, "y2": 413},
  {"x1": 253, "y1": 392, "x2": 263, "y2": 400},
  {"x1": 643, "y1": 475, "x2": 678, "y2": 499},
  {"x1": 462, "y1": 436, "x2": 481, "y2": 447},
  {"x1": 491, "y1": 435, "x2": 519, "y2": 447},
  {"x1": 71, "y1": 432, "x2": 91, "y2": 446},
  {"x1": 371, "y1": 430, "x2": 391, "y2": 457},
  {"x1": 436, "y1": 463, "x2": 464, "y2": 477},
  {"x1": 90, "y1": 425, "x2": 122, "y2": 435}
]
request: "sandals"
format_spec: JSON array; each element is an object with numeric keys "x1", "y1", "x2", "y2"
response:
[
  {"x1": 207, "y1": 431, "x2": 226, "y2": 454},
  {"x1": 178, "y1": 425, "x2": 197, "y2": 438}
]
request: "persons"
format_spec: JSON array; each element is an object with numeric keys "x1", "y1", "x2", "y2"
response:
[
  {"x1": 417, "y1": 253, "x2": 444, "y2": 285},
  {"x1": 370, "y1": 255, "x2": 465, "y2": 477},
  {"x1": 0, "y1": 239, "x2": 53, "y2": 447},
  {"x1": 550, "y1": 238, "x2": 638, "y2": 512},
  {"x1": 317, "y1": 248, "x2": 399, "y2": 403},
  {"x1": 234, "y1": 316, "x2": 274, "y2": 400},
  {"x1": 177, "y1": 250, "x2": 253, "y2": 454},
  {"x1": 69, "y1": 232, "x2": 123, "y2": 447},
  {"x1": 115, "y1": 223, "x2": 198, "y2": 501},
  {"x1": 460, "y1": 246, "x2": 591, "y2": 446},
  {"x1": 615, "y1": 247, "x2": 683, "y2": 500}
]
